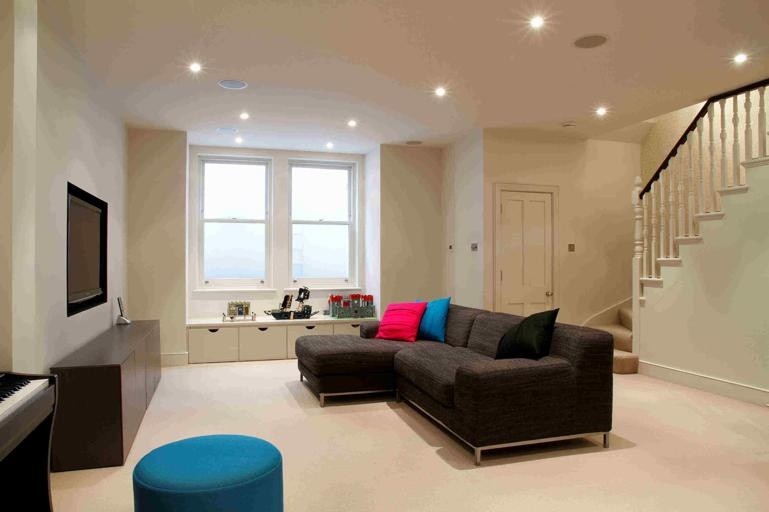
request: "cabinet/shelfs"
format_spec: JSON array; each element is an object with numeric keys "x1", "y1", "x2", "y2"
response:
[
  {"x1": 187, "y1": 313, "x2": 377, "y2": 365},
  {"x1": 48, "y1": 318, "x2": 162, "y2": 477}
]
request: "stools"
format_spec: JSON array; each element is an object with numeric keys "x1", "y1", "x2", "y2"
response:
[{"x1": 134, "y1": 432, "x2": 285, "y2": 511}]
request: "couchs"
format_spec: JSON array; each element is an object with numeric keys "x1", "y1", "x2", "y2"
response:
[{"x1": 298, "y1": 297, "x2": 615, "y2": 465}]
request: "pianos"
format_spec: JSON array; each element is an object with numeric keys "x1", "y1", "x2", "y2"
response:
[{"x1": 0, "y1": 369, "x2": 60, "y2": 512}]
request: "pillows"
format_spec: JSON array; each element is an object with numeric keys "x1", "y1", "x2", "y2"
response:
[
  {"x1": 376, "y1": 296, "x2": 453, "y2": 344},
  {"x1": 496, "y1": 307, "x2": 560, "y2": 359}
]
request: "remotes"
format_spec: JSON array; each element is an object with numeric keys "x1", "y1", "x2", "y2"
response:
[{"x1": 117, "y1": 296, "x2": 126, "y2": 318}]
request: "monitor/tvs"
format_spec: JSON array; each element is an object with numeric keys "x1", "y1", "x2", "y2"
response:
[{"x1": 66, "y1": 193, "x2": 104, "y2": 304}]
